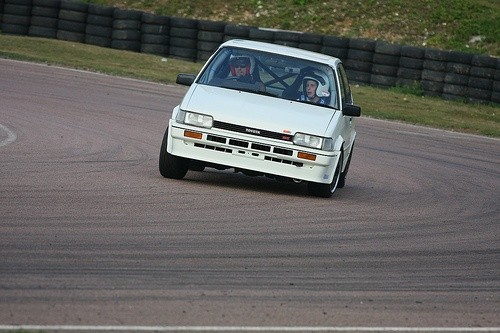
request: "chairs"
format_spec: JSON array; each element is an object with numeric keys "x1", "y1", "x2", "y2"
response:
[{"x1": 281, "y1": 67, "x2": 331, "y2": 107}]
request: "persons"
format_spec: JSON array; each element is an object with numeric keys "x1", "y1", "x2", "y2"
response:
[
  {"x1": 229, "y1": 51, "x2": 267, "y2": 90},
  {"x1": 296, "y1": 69, "x2": 330, "y2": 105}
]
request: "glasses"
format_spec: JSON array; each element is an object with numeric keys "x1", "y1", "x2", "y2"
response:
[{"x1": 233, "y1": 62, "x2": 248, "y2": 68}]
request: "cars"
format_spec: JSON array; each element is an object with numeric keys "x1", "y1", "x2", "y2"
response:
[{"x1": 158, "y1": 39, "x2": 362, "y2": 198}]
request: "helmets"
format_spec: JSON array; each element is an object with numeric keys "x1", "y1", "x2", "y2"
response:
[
  {"x1": 302, "y1": 70, "x2": 330, "y2": 97},
  {"x1": 229, "y1": 54, "x2": 255, "y2": 75}
]
